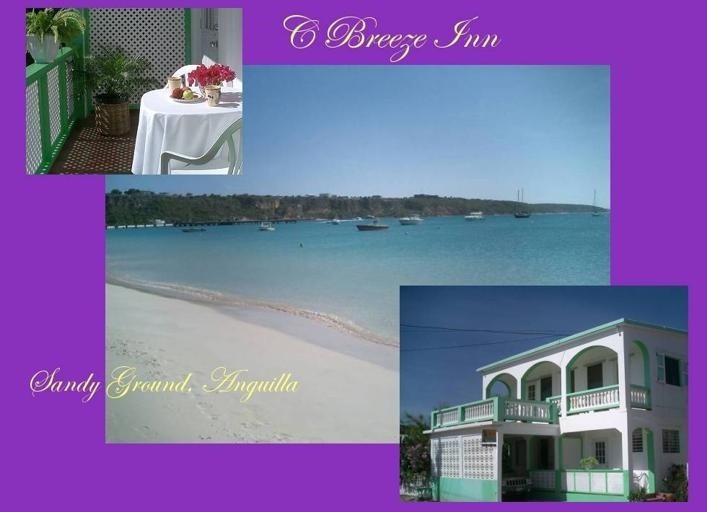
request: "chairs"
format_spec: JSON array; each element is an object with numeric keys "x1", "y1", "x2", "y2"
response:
[
  {"x1": 164, "y1": 64, "x2": 228, "y2": 88},
  {"x1": 161, "y1": 117, "x2": 243, "y2": 175}
]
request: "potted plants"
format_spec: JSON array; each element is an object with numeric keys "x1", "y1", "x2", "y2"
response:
[
  {"x1": 67, "y1": 44, "x2": 157, "y2": 137},
  {"x1": 26, "y1": 8, "x2": 86, "y2": 64}
]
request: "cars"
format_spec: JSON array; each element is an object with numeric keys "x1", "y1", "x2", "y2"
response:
[{"x1": 502, "y1": 459, "x2": 534, "y2": 499}]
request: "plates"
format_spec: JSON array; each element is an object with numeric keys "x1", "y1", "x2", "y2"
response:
[{"x1": 170, "y1": 92, "x2": 205, "y2": 105}]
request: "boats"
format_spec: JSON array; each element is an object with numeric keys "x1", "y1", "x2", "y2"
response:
[
  {"x1": 181, "y1": 226, "x2": 206, "y2": 233},
  {"x1": 398, "y1": 217, "x2": 423, "y2": 226},
  {"x1": 257, "y1": 221, "x2": 275, "y2": 231},
  {"x1": 357, "y1": 221, "x2": 389, "y2": 231},
  {"x1": 465, "y1": 212, "x2": 484, "y2": 221},
  {"x1": 515, "y1": 211, "x2": 530, "y2": 219}
]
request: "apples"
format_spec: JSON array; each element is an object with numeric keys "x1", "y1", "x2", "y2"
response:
[{"x1": 172, "y1": 87, "x2": 193, "y2": 100}]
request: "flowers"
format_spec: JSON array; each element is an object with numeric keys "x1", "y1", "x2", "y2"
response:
[{"x1": 186, "y1": 64, "x2": 236, "y2": 87}]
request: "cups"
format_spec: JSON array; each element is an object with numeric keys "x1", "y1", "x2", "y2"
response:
[
  {"x1": 168, "y1": 76, "x2": 182, "y2": 96},
  {"x1": 227, "y1": 80, "x2": 233, "y2": 88}
]
